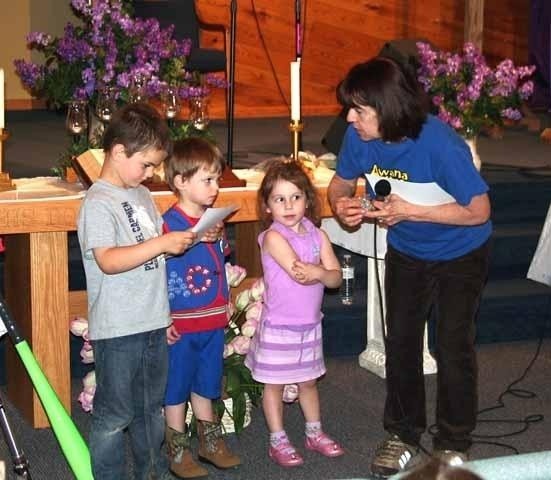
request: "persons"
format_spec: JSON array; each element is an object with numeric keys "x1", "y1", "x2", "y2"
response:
[
  {"x1": 244, "y1": 159, "x2": 347, "y2": 468},
  {"x1": 77, "y1": 100, "x2": 226, "y2": 479},
  {"x1": 155, "y1": 135, "x2": 245, "y2": 480},
  {"x1": 326, "y1": 55, "x2": 496, "y2": 480}
]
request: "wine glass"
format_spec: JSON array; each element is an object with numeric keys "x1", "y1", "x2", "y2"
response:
[{"x1": 379, "y1": 218, "x2": 385, "y2": 224}]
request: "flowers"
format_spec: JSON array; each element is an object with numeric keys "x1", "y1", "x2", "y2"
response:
[{"x1": 375, "y1": 180, "x2": 391, "y2": 201}]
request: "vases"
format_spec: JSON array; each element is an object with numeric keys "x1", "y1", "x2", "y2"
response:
[
  {"x1": 68, "y1": 260, "x2": 262, "y2": 441},
  {"x1": 413, "y1": 39, "x2": 538, "y2": 137},
  {"x1": 13, "y1": 0, "x2": 238, "y2": 121}
]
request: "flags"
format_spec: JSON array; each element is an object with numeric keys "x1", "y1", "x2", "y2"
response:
[
  {"x1": 463, "y1": 135, "x2": 482, "y2": 173},
  {"x1": 148, "y1": 394, "x2": 252, "y2": 438}
]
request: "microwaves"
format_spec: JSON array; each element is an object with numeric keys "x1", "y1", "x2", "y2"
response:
[{"x1": 0, "y1": 302, "x2": 91, "y2": 476}]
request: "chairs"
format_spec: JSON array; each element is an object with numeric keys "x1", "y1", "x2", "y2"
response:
[
  {"x1": 370, "y1": 433, "x2": 421, "y2": 478},
  {"x1": 430, "y1": 444, "x2": 469, "y2": 466}
]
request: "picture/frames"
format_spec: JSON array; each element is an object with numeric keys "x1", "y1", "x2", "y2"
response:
[{"x1": 0, "y1": 161, "x2": 368, "y2": 430}]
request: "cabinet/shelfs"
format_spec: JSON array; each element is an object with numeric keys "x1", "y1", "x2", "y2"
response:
[{"x1": 339, "y1": 254, "x2": 355, "y2": 305}]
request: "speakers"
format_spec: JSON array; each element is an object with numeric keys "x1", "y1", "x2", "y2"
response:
[
  {"x1": 193, "y1": 413, "x2": 241, "y2": 470},
  {"x1": 161, "y1": 421, "x2": 210, "y2": 479}
]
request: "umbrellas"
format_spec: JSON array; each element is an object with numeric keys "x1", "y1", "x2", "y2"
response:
[
  {"x1": 268, "y1": 437, "x2": 304, "y2": 470},
  {"x1": 304, "y1": 429, "x2": 344, "y2": 458}
]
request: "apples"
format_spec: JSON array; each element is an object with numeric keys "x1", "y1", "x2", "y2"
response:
[{"x1": 290, "y1": 61, "x2": 301, "y2": 121}]
